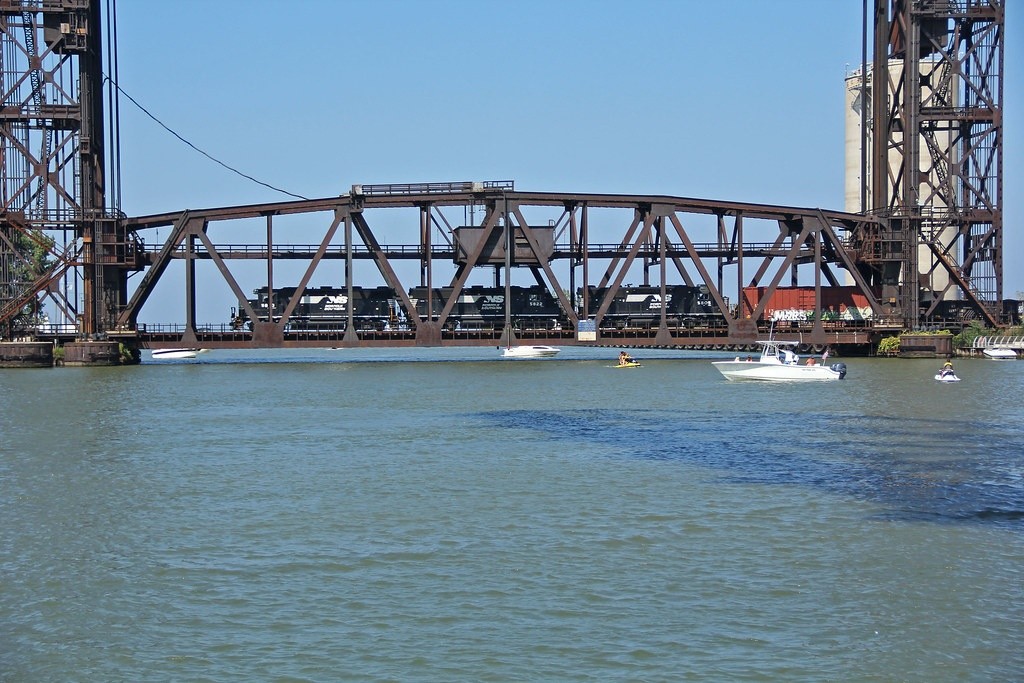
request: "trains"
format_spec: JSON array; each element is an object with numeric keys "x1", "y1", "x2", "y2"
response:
[{"x1": 225, "y1": 280, "x2": 948, "y2": 337}]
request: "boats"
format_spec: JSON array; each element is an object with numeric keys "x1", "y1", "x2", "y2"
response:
[
  {"x1": 982, "y1": 348, "x2": 1017, "y2": 363},
  {"x1": 614, "y1": 363, "x2": 641, "y2": 368},
  {"x1": 710, "y1": 340, "x2": 847, "y2": 382},
  {"x1": 934, "y1": 369, "x2": 964, "y2": 384},
  {"x1": 151, "y1": 347, "x2": 197, "y2": 359},
  {"x1": 500, "y1": 346, "x2": 562, "y2": 358}
]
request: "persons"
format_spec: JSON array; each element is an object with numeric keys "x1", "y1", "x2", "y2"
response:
[
  {"x1": 619, "y1": 351, "x2": 630, "y2": 360},
  {"x1": 778, "y1": 346, "x2": 796, "y2": 364},
  {"x1": 821, "y1": 349, "x2": 829, "y2": 366},
  {"x1": 944, "y1": 359, "x2": 953, "y2": 370},
  {"x1": 806, "y1": 355, "x2": 816, "y2": 366}
]
